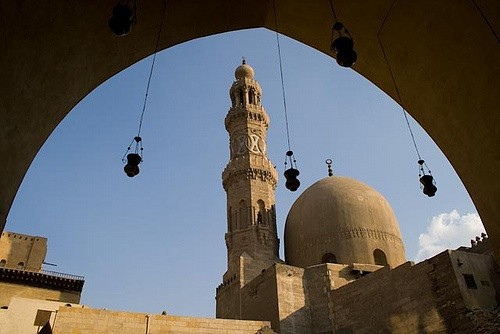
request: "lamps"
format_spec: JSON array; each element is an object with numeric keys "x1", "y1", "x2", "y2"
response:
[
  {"x1": 271, "y1": 2, "x2": 300, "y2": 191},
  {"x1": 120, "y1": 1, "x2": 169, "y2": 176},
  {"x1": 377, "y1": 36, "x2": 435, "y2": 195},
  {"x1": 107, "y1": 0, "x2": 134, "y2": 37},
  {"x1": 329, "y1": 1, "x2": 359, "y2": 67}
]
